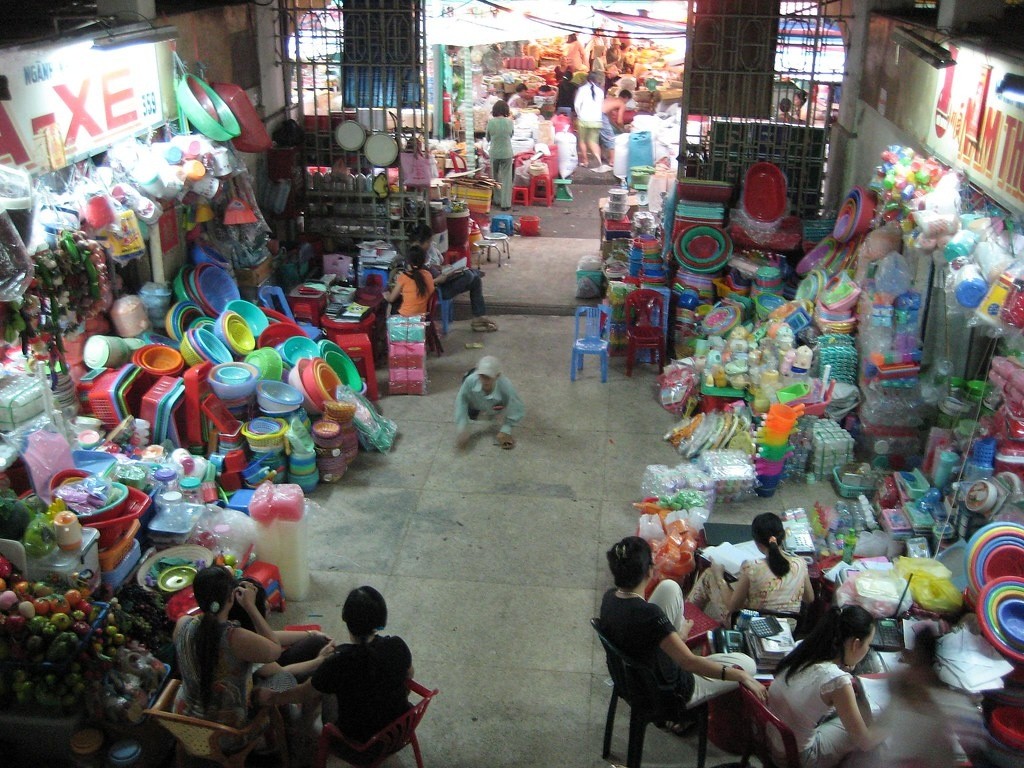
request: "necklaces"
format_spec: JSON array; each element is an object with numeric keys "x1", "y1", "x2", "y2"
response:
[{"x1": 616, "y1": 588, "x2": 647, "y2": 602}]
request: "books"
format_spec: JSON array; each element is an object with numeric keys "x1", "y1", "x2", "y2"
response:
[
  {"x1": 702, "y1": 541, "x2": 766, "y2": 576},
  {"x1": 743, "y1": 617, "x2": 797, "y2": 672}
]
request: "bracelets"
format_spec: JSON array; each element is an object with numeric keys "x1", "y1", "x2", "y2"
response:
[{"x1": 722, "y1": 664, "x2": 729, "y2": 681}]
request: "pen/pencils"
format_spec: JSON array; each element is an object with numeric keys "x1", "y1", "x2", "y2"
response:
[{"x1": 764, "y1": 638, "x2": 781, "y2": 643}]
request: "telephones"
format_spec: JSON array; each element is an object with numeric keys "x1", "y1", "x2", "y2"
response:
[
  {"x1": 716, "y1": 628, "x2": 746, "y2": 654},
  {"x1": 781, "y1": 521, "x2": 816, "y2": 553}
]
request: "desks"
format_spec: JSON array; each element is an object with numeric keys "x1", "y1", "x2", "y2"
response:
[{"x1": 702, "y1": 522, "x2": 834, "y2": 603}]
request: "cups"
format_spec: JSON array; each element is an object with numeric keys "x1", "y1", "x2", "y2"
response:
[
  {"x1": 961, "y1": 214, "x2": 974, "y2": 229},
  {"x1": 870, "y1": 145, "x2": 946, "y2": 221},
  {"x1": 118, "y1": 134, "x2": 234, "y2": 199}
]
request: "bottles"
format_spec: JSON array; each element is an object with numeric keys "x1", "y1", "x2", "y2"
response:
[
  {"x1": 155, "y1": 467, "x2": 204, "y2": 525},
  {"x1": 976, "y1": 272, "x2": 1012, "y2": 325},
  {"x1": 313, "y1": 172, "x2": 374, "y2": 192},
  {"x1": 214, "y1": 524, "x2": 234, "y2": 552},
  {"x1": 469, "y1": 230, "x2": 483, "y2": 253},
  {"x1": 843, "y1": 529, "x2": 855, "y2": 565}
]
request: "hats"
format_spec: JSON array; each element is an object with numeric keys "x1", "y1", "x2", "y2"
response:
[
  {"x1": 611, "y1": 38, "x2": 621, "y2": 46},
  {"x1": 477, "y1": 356, "x2": 503, "y2": 378}
]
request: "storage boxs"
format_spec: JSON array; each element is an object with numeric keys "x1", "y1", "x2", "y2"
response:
[
  {"x1": 598, "y1": 196, "x2": 639, "y2": 261},
  {"x1": 483, "y1": 76, "x2": 523, "y2": 101},
  {"x1": 451, "y1": 185, "x2": 492, "y2": 213},
  {"x1": 233, "y1": 250, "x2": 274, "y2": 307}
]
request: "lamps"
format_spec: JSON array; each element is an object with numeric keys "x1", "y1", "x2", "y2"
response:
[
  {"x1": 889, "y1": 26, "x2": 958, "y2": 70},
  {"x1": 90, "y1": 24, "x2": 182, "y2": 54}
]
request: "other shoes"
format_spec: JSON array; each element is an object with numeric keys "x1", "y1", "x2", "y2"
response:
[
  {"x1": 499, "y1": 434, "x2": 514, "y2": 450},
  {"x1": 471, "y1": 321, "x2": 497, "y2": 332},
  {"x1": 501, "y1": 207, "x2": 511, "y2": 211},
  {"x1": 580, "y1": 162, "x2": 588, "y2": 168}
]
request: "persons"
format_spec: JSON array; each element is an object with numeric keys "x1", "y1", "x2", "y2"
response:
[
  {"x1": 486, "y1": 100, "x2": 514, "y2": 211},
  {"x1": 381, "y1": 225, "x2": 498, "y2": 333},
  {"x1": 598, "y1": 89, "x2": 632, "y2": 167},
  {"x1": 170, "y1": 565, "x2": 336, "y2": 768},
  {"x1": 598, "y1": 536, "x2": 769, "y2": 720},
  {"x1": 573, "y1": 70, "x2": 605, "y2": 167},
  {"x1": 777, "y1": 90, "x2": 807, "y2": 124},
  {"x1": 710, "y1": 512, "x2": 815, "y2": 634},
  {"x1": 557, "y1": 70, "x2": 578, "y2": 112},
  {"x1": 451, "y1": 354, "x2": 527, "y2": 450},
  {"x1": 765, "y1": 605, "x2": 913, "y2": 768},
  {"x1": 506, "y1": 83, "x2": 556, "y2": 120},
  {"x1": 249, "y1": 586, "x2": 415, "y2": 764}
]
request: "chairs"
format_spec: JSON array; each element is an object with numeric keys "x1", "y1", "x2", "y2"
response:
[
  {"x1": 624, "y1": 290, "x2": 666, "y2": 377},
  {"x1": 143, "y1": 676, "x2": 439, "y2": 768},
  {"x1": 570, "y1": 307, "x2": 612, "y2": 384},
  {"x1": 591, "y1": 548, "x2": 806, "y2": 768},
  {"x1": 417, "y1": 286, "x2": 460, "y2": 355}
]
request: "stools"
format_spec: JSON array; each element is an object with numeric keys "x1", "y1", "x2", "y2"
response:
[
  {"x1": 511, "y1": 173, "x2": 554, "y2": 207},
  {"x1": 225, "y1": 489, "x2": 257, "y2": 517},
  {"x1": 361, "y1": 267, "x2": 390, "y2": 290},
  {"x1": 286, "y1": 282, "x2": 328, "y2": 328},
  {"x1": 445, "y1": 214, "x2": 514, "y2": 275},
  {"x1": 336, "y1": 332, "x2": 379, "y2": 401},
  {"x1": 242, "y1": 562, "x2": 285, "y2": 620}
]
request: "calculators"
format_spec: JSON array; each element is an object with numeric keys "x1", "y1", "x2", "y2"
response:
[{"x1": 750, "y1": 614, "x2": 783, "y2": 638}]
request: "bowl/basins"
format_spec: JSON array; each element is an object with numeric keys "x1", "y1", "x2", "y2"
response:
[
  {"x1": 964, "y1": 522, "x2": 1024, "y2": 750},
  {"x1": 750, "y1": 266, "x2": 795, "y2": 321},
  {"x1": 670, "y1": 179, "x2": 742, "y2": 360},
  {"x1": 289, "y1": 452, "x2": 320, "y2": 492},
  {"x1": 44, "y1": 360, "x2": 102, "y2": 449},
  {"x1": 795, "y1": 184, "x2": 875, "y2": 309},
  {"x1": 50, "y1": 469, "x2": 129, "y2": 522},
  {"x1": 177, "y1": 73, "x2": 241, "y2": 142},
  {"x1": 137, "y1": 544, "x2": 213, "y2": 594},
  {"x1": 132, "y1": 246, "x2": 367, "y2": 418},
  {"x1": 642, "y1": 239, "x2": 666, "y2": 286}
]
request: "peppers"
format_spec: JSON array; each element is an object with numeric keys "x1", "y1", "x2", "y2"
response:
[{"x1": 17, "y1": 236, "x2": 100, "y2": 376}]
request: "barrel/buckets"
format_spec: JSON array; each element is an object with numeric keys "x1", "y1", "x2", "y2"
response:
[
  {"x1": 938, "y1": 376, "x2": 996, "y2": 438},
  {"x1": 432, "y1": 210, "x2": 470, "y2": 254},
  {"x1": 54, "y1": 511, "x2": 82, "y2": 551},
  {"x1": 83, "y1": 196, "x2": 148, "y2": 369},
  {"x1": 943, "y1": 230, "x2": 1013, "y2": 308}
]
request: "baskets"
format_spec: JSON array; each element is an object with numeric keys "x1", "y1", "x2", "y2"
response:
[
  {"x1": 88, "y1": 363, "x2": 291, "y2": 496},
  {"x1": 311, "y1": 401, "x2": 358, "y2": 483}
]
